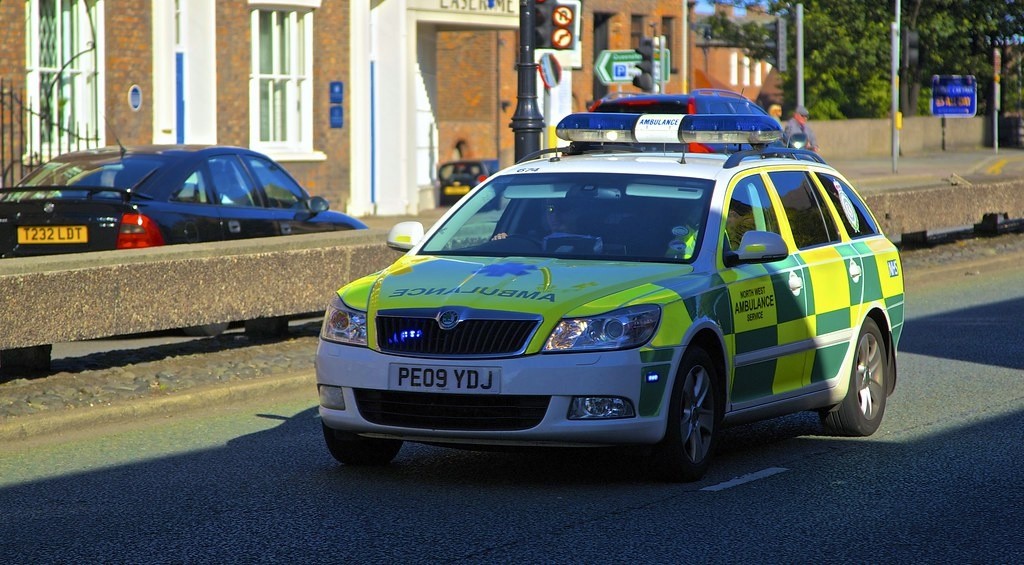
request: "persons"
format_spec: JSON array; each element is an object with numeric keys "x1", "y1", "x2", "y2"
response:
[
  {"x1": 487, "y1": 184, "x2": 591, "y2": 242},
  {"x1": 664, "y1": 206, "x2": 742, "y2": 260},
  {"x1": 767, "y1": 104, "x2": 820, "y2": 155}
]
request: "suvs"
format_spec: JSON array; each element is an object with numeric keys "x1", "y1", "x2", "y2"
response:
[{"x1": 572, "y1": 89, "x2": 812, "y2": 161}]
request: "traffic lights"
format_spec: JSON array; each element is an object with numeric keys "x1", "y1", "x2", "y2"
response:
[
  {"x1": 762, "y1": 17, "x2": 788, "y2": 72},
  {"x1": 630, "y1": 37, "x2": 656, "y2": 93}
]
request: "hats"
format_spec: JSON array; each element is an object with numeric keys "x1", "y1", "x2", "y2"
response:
[{"x1": 797, "y1": 105, "x2": 809, "y2": 116}]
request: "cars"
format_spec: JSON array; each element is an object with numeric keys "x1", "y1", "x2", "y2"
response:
[
  {"x1": 0, "y1": 144, "x2": 370, "y2": 259},
  {"x1": 311, "y1": 111, "x2": 906, "y2": 486}
]
